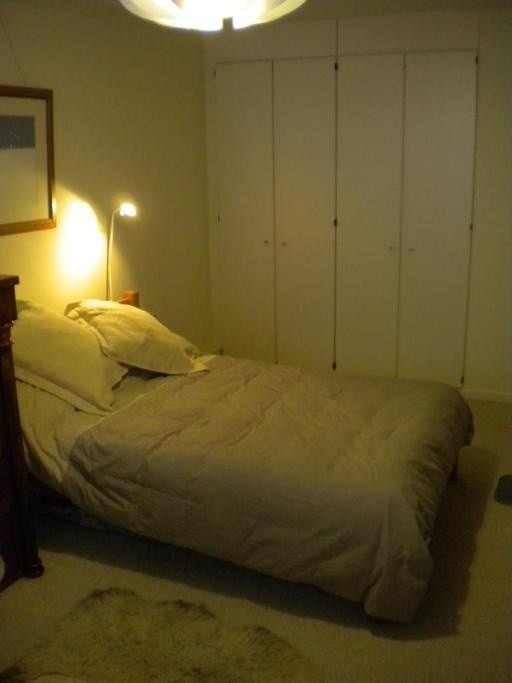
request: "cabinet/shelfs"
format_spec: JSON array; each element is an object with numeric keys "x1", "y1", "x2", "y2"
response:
[{"x1": 0, "y1": 274, "x2": 42, "y2": 592}]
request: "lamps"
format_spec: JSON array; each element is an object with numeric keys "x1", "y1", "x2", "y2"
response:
[{"x1": 108, "y1": 202, "x2": 139, "y2": 300}]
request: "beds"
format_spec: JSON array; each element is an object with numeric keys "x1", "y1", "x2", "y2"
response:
[{"x1": 12, "y1": 276, "x2": 474, "y2": 619}]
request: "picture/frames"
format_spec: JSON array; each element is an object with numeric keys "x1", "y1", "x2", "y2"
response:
[{"x1": 0, "y1": 86, "x2": 60, "y2": 235}]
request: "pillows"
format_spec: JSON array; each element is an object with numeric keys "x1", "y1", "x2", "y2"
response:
[{"x1": 11, "y1": 298, "x2": 208, "y2": 417}]
